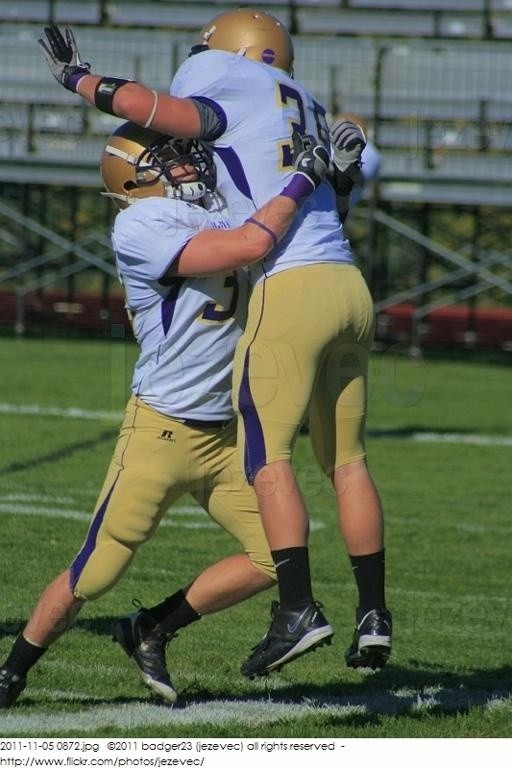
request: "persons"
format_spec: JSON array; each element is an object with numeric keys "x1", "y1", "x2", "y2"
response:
[
  {"x1": 36, "y1": 0, "x2": 397, "y2": 672},
  {"x1": 1, "y1": 103, "x2": 370, "y2": 708}
]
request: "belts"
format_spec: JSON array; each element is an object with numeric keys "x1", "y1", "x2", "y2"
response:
[{"x1": 182, "y1": 419, "x2": 232, "y2": 430}]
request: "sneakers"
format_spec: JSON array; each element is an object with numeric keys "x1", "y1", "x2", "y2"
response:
[
  {"x1": 235, "y1": 596, "x2": 334, "y2": 679},
  {"x1": 109, "y1": 592, "x2": 184, "y2": 707},
  {"x1": 343, "y1": 607, "x2": 396, "y2": 675},
  {"x1": 1, "y1": 668, "x2": 30, "y2": 712}
]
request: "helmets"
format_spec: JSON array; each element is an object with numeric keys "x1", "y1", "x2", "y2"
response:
[
  {"x1": 101, "y1": 111, "x2": 207, "y2": 212},
  {"x1": 192, "y1": 10, "x2": 301, "y2": 84}
]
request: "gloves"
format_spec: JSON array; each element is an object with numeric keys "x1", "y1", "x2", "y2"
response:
[
  {"x1": 327, "y1": 116, "x2": 368, "y2": 194},
  {"x1": 286, "y1": 131, "x2": 338, "y2": 189},
  {"x1": 34, "y1": 22, "x2": 91, "y2": 96}
]
